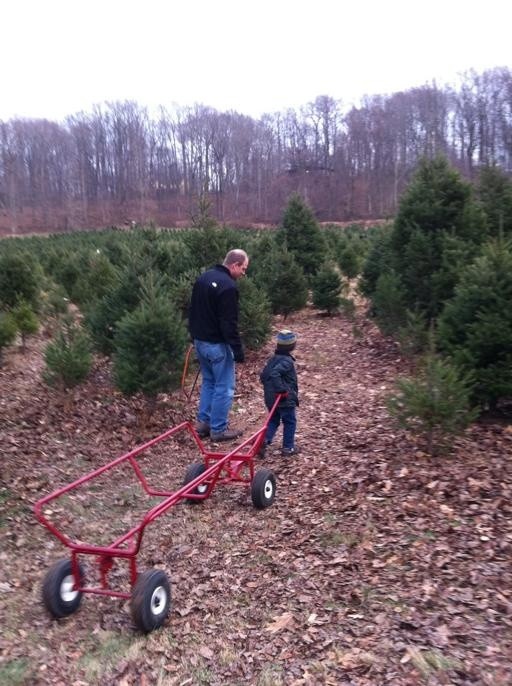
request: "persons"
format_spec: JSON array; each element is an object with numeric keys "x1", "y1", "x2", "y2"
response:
[
  {"x1": 258, "y1": 329, "x2": 302, "y2": 459},
  {"x1": 188, "y1": 249, "x2": 249, "y2": 443}
]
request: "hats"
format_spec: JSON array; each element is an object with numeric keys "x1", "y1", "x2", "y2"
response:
[{"x1": 277, "y1": 330, "x2": 296, "y2": 352}]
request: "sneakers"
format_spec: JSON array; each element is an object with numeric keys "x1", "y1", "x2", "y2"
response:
[
  {"x1": 196, "y1": 420, "x2": 210, "y2": 437},
  {"x1": 210, "y1": 429, "x2": 242, "y2": 442},
  {"x1": 282, "y1": 446, "x2": 300, "y2": 455}
]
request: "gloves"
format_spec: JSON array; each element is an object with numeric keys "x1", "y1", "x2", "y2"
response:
[
  {"x1": 276, "y1": 392, "x2": 288, "y2": 401},
  {"x1": 233, "y1": 348, "x2": 244, "y2": 363}
]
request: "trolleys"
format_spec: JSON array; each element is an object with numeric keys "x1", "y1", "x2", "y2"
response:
[{"x1": 35, "y1": 395, "x2": 286, "y2": 634}]
